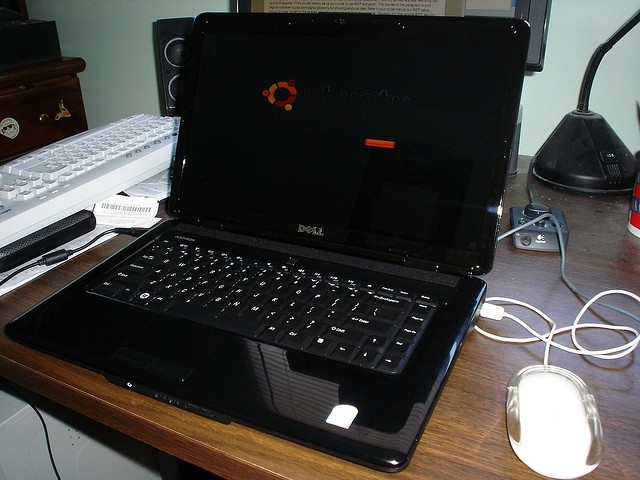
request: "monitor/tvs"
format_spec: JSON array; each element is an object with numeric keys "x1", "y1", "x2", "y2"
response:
[{"x1": 167, "y1": 14, "x2": 530, "y2": 275}]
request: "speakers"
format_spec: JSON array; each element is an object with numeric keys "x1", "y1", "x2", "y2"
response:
[{"x1": 150, "y1": 16, "x2": 195, "y2": 117}]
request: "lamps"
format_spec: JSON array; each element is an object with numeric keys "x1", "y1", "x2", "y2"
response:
[{"x1": 530, "y1": 9, "x2": 640, "y2": 195}]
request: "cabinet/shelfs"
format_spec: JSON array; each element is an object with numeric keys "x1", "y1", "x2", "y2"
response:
[{"x1": 0, "y1": 56, "x2": 89, "y2": 164}]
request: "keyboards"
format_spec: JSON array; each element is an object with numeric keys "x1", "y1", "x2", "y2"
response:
[
  {"x1": 0, "y1": 114, "x2": 179, "y2": 251},
  {"x1": 86, "y1": 232, "x2": 443, "y2": 377}
]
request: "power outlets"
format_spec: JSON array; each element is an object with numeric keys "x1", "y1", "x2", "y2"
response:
[{"x1": 509, "y1": 206, "x2": 569, "y2": 252}]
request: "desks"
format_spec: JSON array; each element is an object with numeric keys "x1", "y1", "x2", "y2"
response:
[{"x1": 1, "y1": 153, "x2": 640, "y2": 479}]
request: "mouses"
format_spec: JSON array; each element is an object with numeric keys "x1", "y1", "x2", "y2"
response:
[{"x1": 505, "y1": 364, "x2": 605, "y2": 476}]
